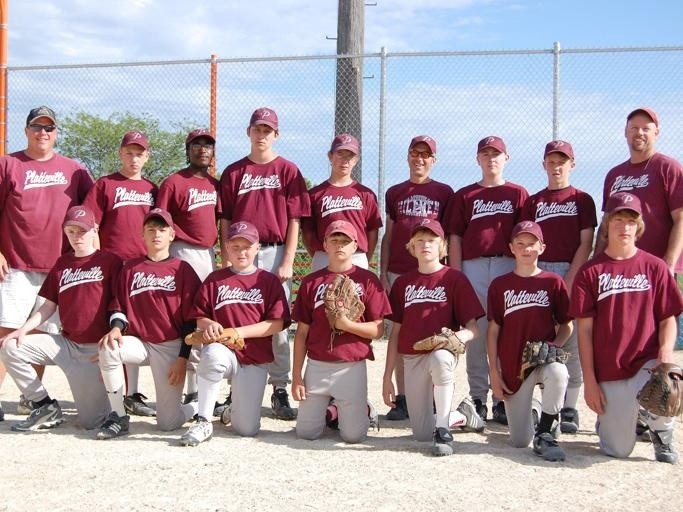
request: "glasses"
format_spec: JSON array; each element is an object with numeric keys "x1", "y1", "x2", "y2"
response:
[
  {"x1": 409, "y1": 150, "x2": 434, "y2": 159},
  {"x1": 191, "y1": 143, "x2": 216, "y2": 153},
  {"x1": 29, "y1": 124, "x2": 56, "y2": 132}
]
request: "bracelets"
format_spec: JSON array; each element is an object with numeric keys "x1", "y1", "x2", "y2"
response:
[{"x1": 379, "y1": 271, "x2": 388, "y2": 276}]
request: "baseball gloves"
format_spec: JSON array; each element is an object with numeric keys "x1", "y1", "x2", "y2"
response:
[
  {"x1": 185, "y1": 328, "x2": 244, "y2": 350},
  {"x1": 521, "y1": 341, "x2": 568, "y2": 379},
  {"x1": 637, "y1": 363, "x2": 682, "y2": 417},
  {"x1": 413, "y1": 327, "x2": 467, "y2": 354},
  {"x1": 323, "y1": 273, "x2": 365, "y2": 334}
]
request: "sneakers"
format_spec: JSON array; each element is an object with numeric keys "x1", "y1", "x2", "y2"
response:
[
  {"x1": 330, "y1": 399, "x2": 338, "y2": 430},
  {"x1": 367, "y1": 398, "x2": 378, "y2": 429},
  {"x1": 457, "y1": 397, "x2": 488, "y2": 433},
  {"x1": 595, "y1": 409, "x2": 653, "y2": 443},
  {"x1": 492, "y1": 400, "x2": 508, "y2": 425},
  {"x1": 96, "y1": 411, "x2": 129, "y2": 439},
  {"x1": 11, "y1": 399, "x2": 64, "y2": 431},
  {"x1": 533, "y1": 433, "x2": 566, "y2": 461},
  {"x1": 17, "y1": 395, "x2": 36, "y2": 415},
  {"x1": 648, "y1": 416, "x2": 678, "y2": 463},
  {"x1": 270, "y1": 385, "x2": 295, "y2": 420},
  {"x1": 531, "y1": 396, "x2": 580, "y2": 437},
  {"x1": 183, "y1": 391, "x2": 199, "y2": 418},
  {"x1": 431, "y1": 427, "x2": 453, "y2": 456},
  {"x1": 214, "y1": 394, "x2": 231, "y2": 425},
  {"x1": 124, "y1": 393, "x2": 156, "y2": 416},
  {"x1": 0, "y1": 401, "x2": 4, "y2": 421},
  {"x1": 384, "y1": 394, "x2": 409, "y2": 420},
  {"x1": 179, "y1": 417, "x2": 213, "y2": 447}
]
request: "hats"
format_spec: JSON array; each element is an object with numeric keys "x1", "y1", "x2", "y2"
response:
[
  {"x1": 227, "y1": 221, "x2": 259, "y2": 245},
  {"x1": 330, "y1": 133, "x2": 359, "y2": 156},
  {"x1": 326, "y1": 220, "x2": 358, "y2": 242},
  {"x1": 186, "y1": 129, "x2": 216, "y2": 144},
  {"x1": 408, "y1": 134, "x2": 437, "y2": 153},
  {"x1": 626, "y1": 107, "x2": 659, "y2": 127},
  {"x1": 604, "y1": 191, "x2": 641, "y2": 216},
  {"x1": 476, "y1": 136, "x2": 507, "y2": 155},
  {"x1": 121, "y1": 130, "x2": 149, "y2": 149},
  {"x1": 64, "y1": 204, "x2": 95, "y2": 232},
  {"x1": 142, "y1": 208, "x2": 173, "y2": 227},
  {"x1": 511, "y1": 221, "x2": 544, "y2": 242},
  {"x1": 249, "y1": 107, "x2": 278, "y2": 131},
  {"x1": 543, "y1": 140, "x2": 574, "y2": 161},
  {"x1": 26, "y1": 106, "x2": 56, "y2": 127},
  {"x1": 412, "y1": 219, "x2": 444, "y2": 239}
]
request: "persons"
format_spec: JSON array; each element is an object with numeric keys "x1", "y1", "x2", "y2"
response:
[
  {"x1": 487, "y1": 220, "x2": 575, "y2": 462},
  {"x1": 288, "y1": 220, "x2": 393, "y2": 446},
  {"x1": 382, "y1": 218, "x2": 487, "y2": 456},
  {"x1": 179, "y1": 220, "x2": 292, "y2": 448},
  {"x1": 380, "y1": 136, "x2": 455, "y2": 421},
  {"x1": 95, "y1": 208, "x2": 202, "y2": 440},
  {"x1": 0, "y1": 206, "x2": 123, "y2": 432},
  {"x1": 593, "y1": 107, "x2": 683, "y2": 439},
  {"x1": 214, "y1": 107, "x2": 313, "y2": 420},
  {"x1": 78, "y1": 130, "x2": 158, "y2": 417},
  {"x1": 566, "y1": 192, "x2": 678, "y2": 465},
  {"x1": 516, "y1": 140, "x2": 598, "y2": 433},
  {"x1": 154, "y1": 128, "x2": 222, "y2": 403},
  {"x1": 440, "y1": 136, "x2": 530, "y2": 426},
  {"x1": 0, "y1": 106, "x2": 93, "y2": 422},
  {"x1": 298, "y1": 134, "x2": 383, "y2": 274}
]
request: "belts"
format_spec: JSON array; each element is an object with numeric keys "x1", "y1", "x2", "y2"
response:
[{"x1": 258, "y1": 241, "x2": 285, "y2": 246}]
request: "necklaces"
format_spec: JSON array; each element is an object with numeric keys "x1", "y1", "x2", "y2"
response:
[{"x1": 643, "y1": 156, "x2": 654, "y2": 176}]
